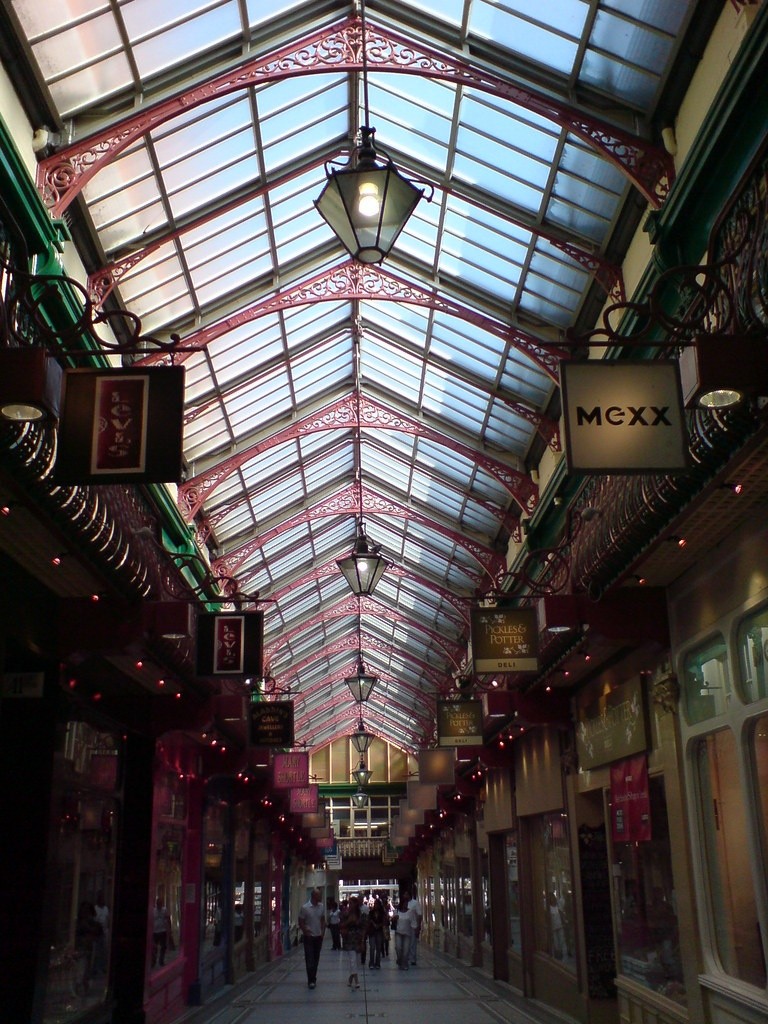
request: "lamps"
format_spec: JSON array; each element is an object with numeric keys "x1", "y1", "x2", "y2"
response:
[
  {"x1": 348, "y1": 701, "x2": 375, "y2": 752},
  {"x1": 537, "y1": 595, "x2": 578, "y2": 635},
  {"x1": 0, "y1": 346, "x2": 63, "y2": 424},
  {"x1": 484, "y1": 691, "x2": 510, "y2": 718},
  {"x1": 155, "y1": 602, "x2": 194, "y2": 639},
  {"x1": 352, "y1": 786, "x2": 368, "y2": 808},
  {"x1": 336, "y1": 338, "x2": 394, "y2": 596},
  {"x1": 255, "y1": 748, "x2": 269, "y2": 767},
  {"x1": 313, "y1": 0, "x2": 434, "y2": 267},
  {"x1": 352, "y1": 752, "x2": 373, "y2": 786},
  {"x1": 455, "y1": 746, "x2": 473, "y2": 762},
  {"x1": 679, "y1": 334, "x2": 747, "y2": 413},
  {"x1": 221, "y1": 696, "x2": 242, "y2": 721},
  {"x1": 344, "y1": 596, "x2": 379, "y2": 703}
]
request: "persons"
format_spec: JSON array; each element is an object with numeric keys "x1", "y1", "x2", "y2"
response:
[
  {"x1": 95, "y1": 897, "x2": 109, "y2": 940},
  {"x1": 339, "y1": 897, "x2": 365, "y2": 988},
  {"x1": 368, "y1": 899, "x2": 393, "y2": 970},
  {"x1": 328, "y1": 896, "x2": 390, "y2": 958},
  {"x1": 73, "y1": 900, "x2": 108, "y2": 1010},
  {"x1": 150, "y1": 899, "x2": 171, "y2": 968},
  {"x1": 298, "y1": 888, "x2": 326, "y2": 989},
  {"x1": 401, "y1": 890, "x2": 423, "y2": 965},
  {"x1": 392, "y1": 899, "x2": 418, "y2": 970}
]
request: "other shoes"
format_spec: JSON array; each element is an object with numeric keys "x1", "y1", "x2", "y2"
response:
[
  {"x1": 347, "y1": 981, "x2": 351, "y2": 986},
  {"x1": 355, "y1": 982, "x2": 360, "y2": 988},
  {"x1": 375, "y1": 965, "x2": 380, "y2": 969},
  {"x1": 310, "y1": 982, "x2": 316, "y2": 988},
  {"x1": 370, "y1": 965, "x2": 374, "y2": 969},
  {"x1": 398, "y1": 965, "x2": 408, "y2": 970},
  {"x1": 408, "y1": 960, "x2": 416, "y2": 965}
]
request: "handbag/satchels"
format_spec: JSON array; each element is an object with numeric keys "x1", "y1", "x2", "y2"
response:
[
  {"x1": 341, "y1": 913, "x2": 348, "y2": 938},
  {"x1": 391, "y1": 909, "x2": 399, "y2": 930}
]
